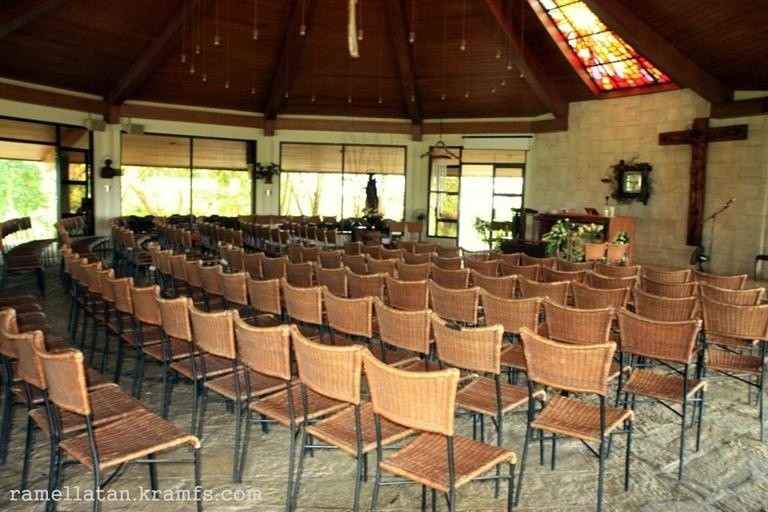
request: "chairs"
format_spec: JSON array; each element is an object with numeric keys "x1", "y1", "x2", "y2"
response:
[
  {"x1": 490, "y1": 250, "x2": 520, "y2": 262},
  {"x1": 463, "y1": 255, "x2": 499, "y2": 275},
  {"x1": 521, "y1": 252, "x2": 555, "y2": 265},
  {"x1": 428, "y1": 309, "x2": 550, "y2": 498},
  {"x1": 166, "y1": 252, "x2": 186, "y2": 281},
  {"x1": 344, "y1": 240, "x2": 361, "y2": 254},
  {"x1": 393, "y1": 258, "x2": 429, "y2": 281},
  {"x1": 641, "y1": 266, "x2": 691, "y2": 283},
  {"x1": 699, "y1": 294, "x2": 768, "y2": 442},
  {"x1": 568, "y1": 280, "x2": 631, "y2": 309},
  {"x1": 181, "y1": 258, "x2": 199, "y2": 283},
  {"x1": 692, "y1": 268, "x2": 747, "y2": 291},
  {"x1": 318, "y1": 249, "x2": 341, "y2": 267},
  {"x1": 378, "y1": 244, "x2": 402, "y2": 259},
  {"x1": 216, "y1": 244, "x2": 229, "y2": 262},
  {"x1": 319, "y1": 282, "x2": 421, "y2": 367},
  {"x1": 195, "y1": 261, "x2": 222, "y2": 303},
  {"x1": 187, "y1": 299, "x2": 301, "y2": 483},
  {"x1": 239, "y1": 249, "x2": 262, "y2": 276},
  {"x1": 283, "y1": 257, "x2": 312, "y2": 286},
  {"x1": 151, "y1": 283, "x2": 246, "y2": 436},
  {"x1": 243, "y1": 274, "x2": 280, "y2": 325},
  {"x1": 460, "y1": 246, "x2": 490, "y2": 260},
  {"x1": 516, "y1": 274, "x2": 567, "y2": 304},
  {"x1": 232, "y1": 307, "x2": 356, "y2": 503},
  {"x1": 287, "y1": 241, "x2": 303, "y2": 262},
  {"x1": 402, "y1": 249, "x2": 431, "y2": 264},
  {"x1": 125, "y1": 280, "x2": 200, "y2": 421},
  {"x1": 497, "y1": 259, "x2": 539, "y2": 278},
  {"x1": 53, "y1": 213, "x2": 106, "y2": 313},
  {"x1": 615, "y1": 305, "x2": 708, "y2": 491},
  {"x1": 428, "y1": 277, "x2": 478, "y2": 326},
  {"x1": 365, "y1": 253, "x2": 395, "y2": 273},
  {"x1": 29, "y1": 333, "x2": 202, "y2": 512},
  {"x1": 557, "y1": 256, "x2": 594, "y2": 271},
  {"x1": 344, "y1": 266, "x2": 384, "y2": 297},
  {"x1": 631, "y1": 286, "x2": 699, "y2": 320},
  {"x1": 301, "y1": 246, "x2": 319, "y2": 262},
  {"x1": 371, "y1": 293, "x2": 477, "y2": 383},
  {"x1": 432, "y1": 253, "x2": 461, "y2": 268},
  {"x1": 216, "y1": 264, "x2": 247, "y2": 300},
  {"x1": 542, "y1": 295, "x2": 631, "y2": 408},
  {"x1": 585, "y1": 270, "x2": 639, "y2": 290},
  {"x1": 385, "y1": 272, "x2": 428, "y2": 311},
  {"x1": 314, "y1": 263, "x2": 348, "y2": 295},
  {"x1": 157, "y1": 249, "x2": 172, "y2": 275},
  {"x1": 359, "y1": 244, "x2": 378, "y2": 255},
  {"x1": 518, "y1": 326, "x2": 634, "y2": 512},
  {"x1": 106, "y1": 267, "x2": 147, "y2": 384},
  {"x1": 699, "y1": 278, "x2": 762, "y2": 306},
  {"x1": 146, "y1": 245, "x2": 161, "y2": 268},
  {"x1": 0, "y1": 207, "x2": 50, "y2": 313},
  {"x1": 392, "y1": 238, "x2": 413, "y2": 249},
  {"x1": 258, "y1": 252, "x2": 287, "y2": 281},
  {"x1": 436, "y1": 244, "x2": 459, "y2": 256},
  {"x1": 540, "y1": 264, "x2": 585, "y2": 284},
  {"x1": 341, "y1": 251, "x2": 364, "y2": 272},
  {"x1": 0, "y1": 315, "x2": 162, "y2": 501},
  {"x1": 639, "y1": 277, "x2": 698, "y2": 300},
  {"x1": 227, "y1": 244, "x2": 242, "y2": 271},
  {"x1": 109, "y1": 212, "x2": 340, "y2": 243},
  {"x1": 412, "y1": 241, "x2": 438, "y2": 253},
  {"x1": 469, "y1": 268, "x2": 517, "y2": 297},
  {"x1": 279, "y1": 276, "x2": 355, "y2": 349},
  {"x1": 286, "y1": 321, "x2": 429, "y2": 509},
  {"x1": 592, "y1": 260, "x2": 640, "y2": 277},
  {"x1": 478, "y1": 287, "x2": 545, "y2": 373},
  {"x1": 430, "y1": 263, "x2": 471, "y2": 289},
  {"x1": 360, "y1": 345, "x2": 516, "y2": 512}
]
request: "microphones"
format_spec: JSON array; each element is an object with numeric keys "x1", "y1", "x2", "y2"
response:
[{"x1": 725, "y1": 196, "x2": 737, "y2": 209}]
